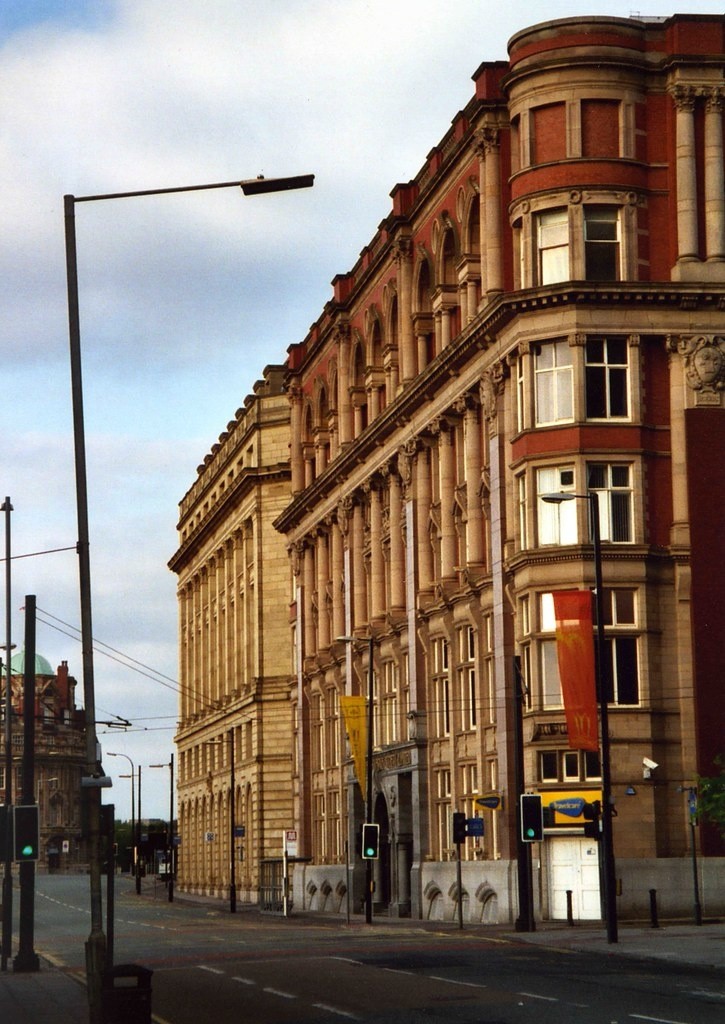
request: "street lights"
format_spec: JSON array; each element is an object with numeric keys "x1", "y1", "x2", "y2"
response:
[
  {"x1": 63, "y1": 174, "x2": 319, "y2": 1023},
  {"x1": 540, "y1": 490, "x2": 618, "y2": 944},
  {"x1": 148, "y1": 753, "x2": 174, "y2": 903},
  {"x1": 335, "y1": 635, "x2": 373, "y2": 925},
  {"x1": 107, "y1": 753, "x2": 140, "y2": 896},
  {"x1": 0, "y1": 495, "x2": 91, "y2": 972},
  {"x1": 202, "y1": 727, "x2": 237, "y2": 913}
]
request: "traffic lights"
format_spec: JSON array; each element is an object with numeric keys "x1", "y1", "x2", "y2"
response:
[
  {"x1": 363, "y1": 824, "x2": 378, "y2": 859},
  {"x1": 453, "y1": 812, "x2": 464, "y2": 842},
  {"x1": 16, "y1": 806, "x2": 40, "y2": 864},
  {"x1": 521, "y1": 796, "x2": 543, "y2": 841}
]
